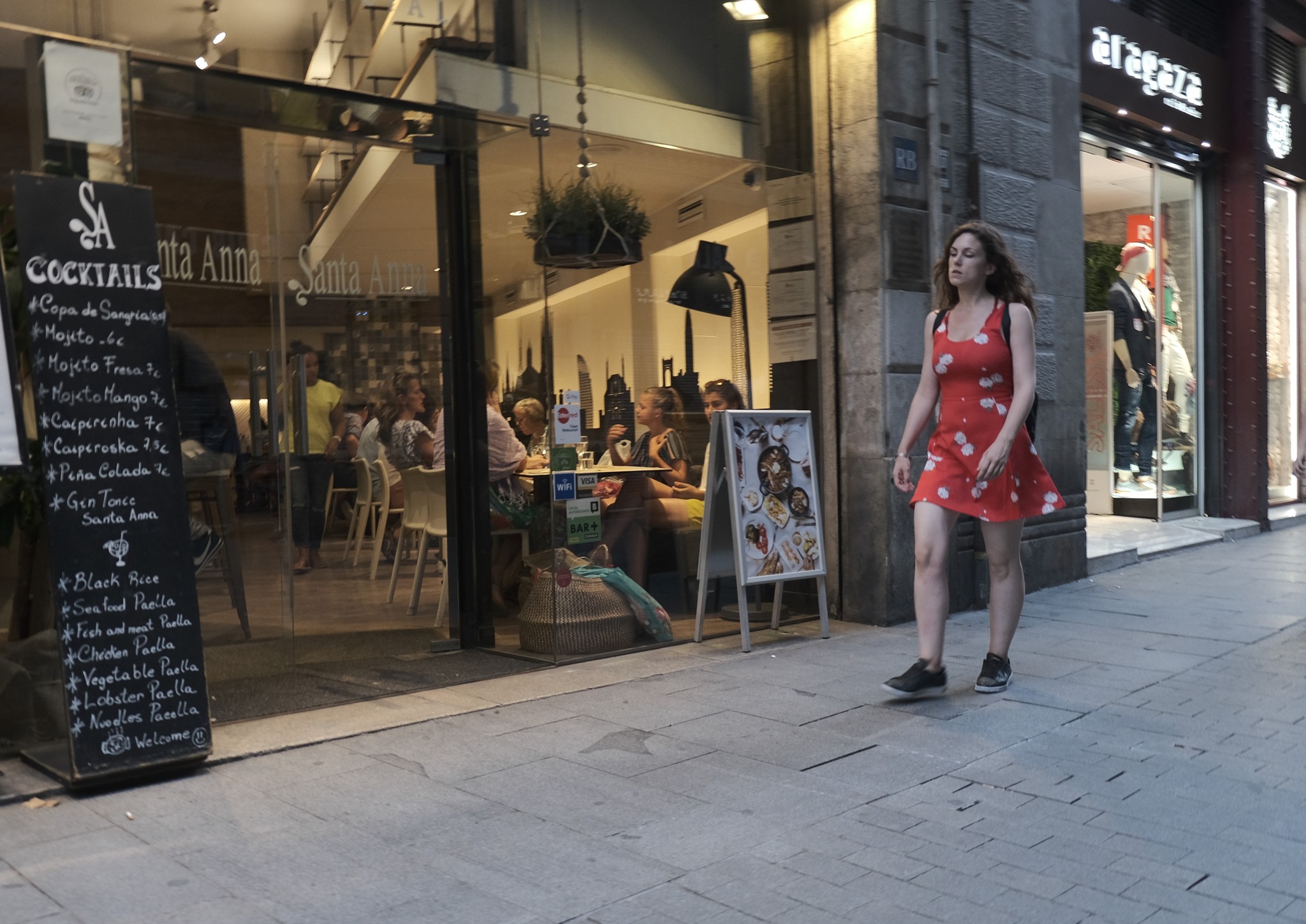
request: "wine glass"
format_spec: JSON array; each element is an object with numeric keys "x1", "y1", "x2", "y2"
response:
[{"x1": 572, "y1": 436, "x2": 589, "y2": 466}]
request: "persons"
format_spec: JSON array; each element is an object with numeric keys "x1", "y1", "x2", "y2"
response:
[
  {"x1": 276, "y1": 345, "x2": 346, "y2": 571},
  {"x1": 167, "y1": 327, "x2": 240, "y2": 577},
  {"x1": 878, "y1": 222, "x2": 1066, "y2": 699},
  {"x1": 231, "y1": 413, "x2": 287, "y2": 514},
  {"x1": 1106, "y1": 242, "x2": 1178, "y2": 495},
  {"x1": 335, "y1": 354, "x2": 746, "y2": 608},
  {"x1": 1151, "y1": 237, "x2": 1195, "y2": 447}
]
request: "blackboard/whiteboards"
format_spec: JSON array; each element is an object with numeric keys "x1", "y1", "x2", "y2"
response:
[{"x1": 16, "y1": 171, "x2": 214, "y2": 780}]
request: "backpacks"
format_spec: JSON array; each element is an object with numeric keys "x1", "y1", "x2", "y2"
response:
[{"x1": 933, "y1": 302, "x2": 1039, "y2": 442}]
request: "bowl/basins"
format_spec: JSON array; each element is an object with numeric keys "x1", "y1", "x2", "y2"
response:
[
  {"x1": 787, "y1": 487, "x2": 809, "y2": 517},
  {"x1": 791, "y1": 534, "x2": 803, "y2": 548}
]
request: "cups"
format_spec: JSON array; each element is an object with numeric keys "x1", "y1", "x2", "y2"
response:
[{"x1": 579, "y1": 451, "x2": 594, "y2": 469}]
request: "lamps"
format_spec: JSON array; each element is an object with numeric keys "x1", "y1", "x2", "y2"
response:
[
  {"x1": 195, "y1": 42, "x2": 222, "y2": 70},
  {"x1": 722, "y1": 0, "x2": 769, "y2": 22},
  {"x1": 666, "y1": 240, "x2": 752, "y2": 409},
  {"x1": 200, "y1": 17, "x2": 226, "y2": 44}
]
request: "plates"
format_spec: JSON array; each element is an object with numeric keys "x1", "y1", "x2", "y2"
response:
[
  {"x1": 742, "y1": 513, "x2": 774, "y2": 559},
  {"x1": 761, "y1": 493, "x2": 790, "y2": 528},
  {"x1": 783, "y1": 424, "x2": 808, "y2": 462},
  {"x1": 773, "y1": 534, "x2": 804, "y2": 572},
  {"x1": 798, "y1": 526, "x2": 819, "y2": 560},
  {"x1": 744, "y1": 487, "x2": 763, "y2": 511}
]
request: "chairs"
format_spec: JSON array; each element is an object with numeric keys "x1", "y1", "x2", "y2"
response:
[{"x1": 324, "y1": 457, "x2": 530, "y2": 628}]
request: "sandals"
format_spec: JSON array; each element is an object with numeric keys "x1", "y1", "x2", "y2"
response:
[{"x1": 490, "y1": 576, "x2": 515, "y2": 618}]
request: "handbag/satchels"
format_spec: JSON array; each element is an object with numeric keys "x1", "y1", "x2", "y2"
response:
[
  {"x1": 517, "y1": 542, "x2": 640, "y2": 654},
  {"x1": 1162, "y1": 400, "x2": 1182, "y2": 438},
  {"x1": 591, "y1": 476, "x2": 625, "y2": 498},
  {"x1": 572, "y1": 562, "x2": 674, "y2": 644},
  {"x1": 597, "y1": 439, "x2": 632, "y2": 466}
]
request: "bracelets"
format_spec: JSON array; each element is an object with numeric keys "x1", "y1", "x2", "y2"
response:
[
  {"x1": 332, "y1": 436, "x2": 342, "y2": 442},
  {"x1": 895, "y1": 452, "x2": 912, "y2": 460}
]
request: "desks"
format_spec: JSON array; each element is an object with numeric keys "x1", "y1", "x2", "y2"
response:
[{"x1": 512, "y1": 465, "x2": 673, "y2": 505}]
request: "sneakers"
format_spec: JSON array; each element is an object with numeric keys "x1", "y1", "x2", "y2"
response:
[
  {"x1": 975, "y1": 652, "x2": 1014, "y2": 693},
  {"x1": 880, "y1": 658, "x2": 948, "y2": 699},
  {"x1": 1137, "y1": 476, "x2": 1178, "y2": 495},
  {"x1": 1115, "y1": 475, "x2": 1154, "y2": 494},
  {"x1": 193, "y1": 527, "x2": 226, "y2": 577}
]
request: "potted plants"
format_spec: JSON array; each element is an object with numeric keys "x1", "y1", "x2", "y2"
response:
[{"x1": 532, "y1": 177, "x2": 650, "y2": 269}]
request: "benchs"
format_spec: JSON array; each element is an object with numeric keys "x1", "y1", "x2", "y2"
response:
[{"x1": 651, "y1": 465, "x2": 702, "y2": 617}]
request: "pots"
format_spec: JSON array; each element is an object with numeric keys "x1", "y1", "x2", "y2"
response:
[{"x1": 757, "y1": 443, "x2": 792, "y2": 496}]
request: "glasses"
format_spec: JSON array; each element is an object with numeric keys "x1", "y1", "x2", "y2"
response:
[
  {"x1": 705, "y1": 379, "x2": 733, "y2": 390},
  {"x1": 514, "y1": 416, "x2": 527, "y2": 425}
]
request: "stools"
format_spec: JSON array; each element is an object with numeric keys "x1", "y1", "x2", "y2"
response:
[{"x1": 188, "y1": 480, "x2": 250, "y2": 642}]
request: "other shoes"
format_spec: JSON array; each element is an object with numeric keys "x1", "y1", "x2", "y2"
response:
[
  {"x1": 308, "y1": 552, "x2": 328, "y2": 569},
  {"x1": 1173, "y1": 432, "x2": 1195, "y2": 447},
  {"x1": 293, "y1": 556, "x2": 312, "y2": 575},
  {"x1": 381, "y1": 525, "x2": 403, "y2": 562}
]
